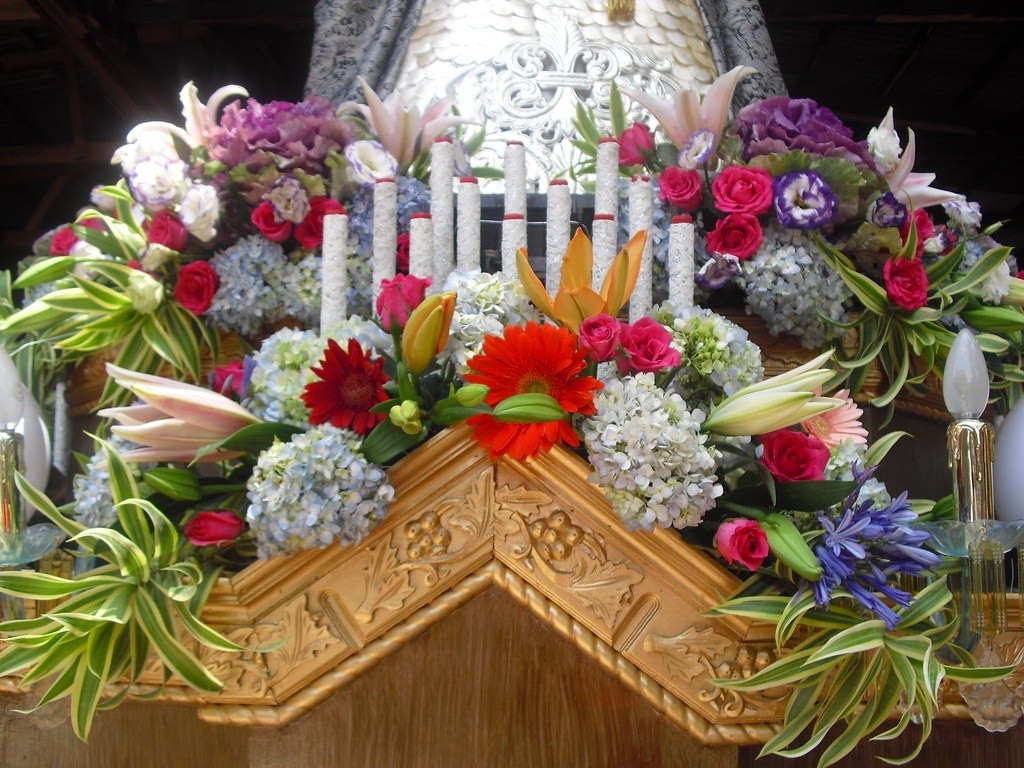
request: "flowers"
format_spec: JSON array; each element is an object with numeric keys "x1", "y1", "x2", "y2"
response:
[{"x1": 0, "y1": 65, "x2": 1024, "y2": 768}]
